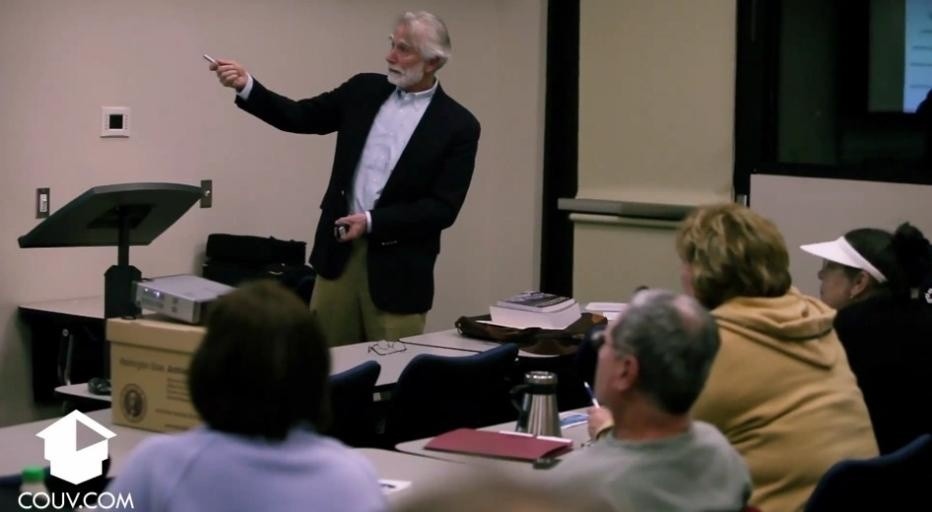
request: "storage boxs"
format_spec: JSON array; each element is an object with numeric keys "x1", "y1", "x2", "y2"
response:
[{"x1": 104, "y1": 314, "x2": 220, "y2": 434}]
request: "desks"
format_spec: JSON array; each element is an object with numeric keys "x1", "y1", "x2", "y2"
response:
[
  {"x1": 399, "y1": 301, "x2": 600, "y2": 410},
  {"x1": 349, "y1": 447, "x2": 480, "y2": 512},
  {"x1": 0, "y1": 340, "x2": 479, "y2": 487},
  {"x1": 394, "y1": 405, "x2": 593, "y2": 466},
  {"x1": 19, "y1": 296, "x2": 155, "y2": 412}
]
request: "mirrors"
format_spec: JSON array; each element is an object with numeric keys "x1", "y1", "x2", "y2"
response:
[{"x1": 754, "y1": 0, "x2": 932, "y2": 185}]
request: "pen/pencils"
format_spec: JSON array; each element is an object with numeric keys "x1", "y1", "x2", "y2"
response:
[
  {"x1": 202, "y1": 52, "x2": 224, "y2": 68},
  {"x1": 584, "y1": 380, "x2": 599, "y2": 410}
]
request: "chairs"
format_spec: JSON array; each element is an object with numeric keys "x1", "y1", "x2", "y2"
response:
[
  {"x1": 797, "y1": 433, "x2": 932, "y2": 512},
  {"x1": 384, "y1": 342, "x2": 518, "y2": 443},
  {"x1": 326, "y1": 361, "x2": 378, "y2": 452}
]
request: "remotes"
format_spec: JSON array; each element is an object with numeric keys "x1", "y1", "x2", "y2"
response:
[{"x1": 333, "y1": 209, "x2": 353, "y2": 241}]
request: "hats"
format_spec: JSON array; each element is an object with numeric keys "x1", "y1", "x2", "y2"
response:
[{"x1": 798, "y1": 228, "x2": 894, "y2": 285}]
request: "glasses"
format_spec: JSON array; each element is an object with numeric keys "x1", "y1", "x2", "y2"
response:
[
  {"x1": 821, "y1": 259, "x2": 859, "y2": 278},
  {"x1": 368, "y1": 338, "x2": 409, "y2": 357}
]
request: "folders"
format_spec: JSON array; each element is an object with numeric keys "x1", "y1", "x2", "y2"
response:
[{"x1": 424, "y1": 426, "x2": 576, "y2": 464}]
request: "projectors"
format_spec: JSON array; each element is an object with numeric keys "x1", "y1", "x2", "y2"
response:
[{"x1": 129, "y1": 273, "x2": 238, "y2": 325}]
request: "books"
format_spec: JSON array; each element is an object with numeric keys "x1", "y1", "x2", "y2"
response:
[
  {"x1": 487, "y1": 287, "x2": 585, "y2": 340},
  {"x1": 587, "y1": 297, "x2": 635, "y2": 319}
]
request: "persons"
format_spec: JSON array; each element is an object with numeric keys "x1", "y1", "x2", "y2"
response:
[
  {"x1": 661, "y1": 204, "x2": 883, "y2": 512},
  {"x1": 208, "y1": 9, "x2": 484, "y2": 343},
  {"x1": 534, "y1": 291, "x2": 752, "y2": 511},
  {"x1": 84, "y1": 281, "x2": 396, "y2": 511},
  {"x1": 798, "y1": 223, "x2": 931, "y2": 454}
]
